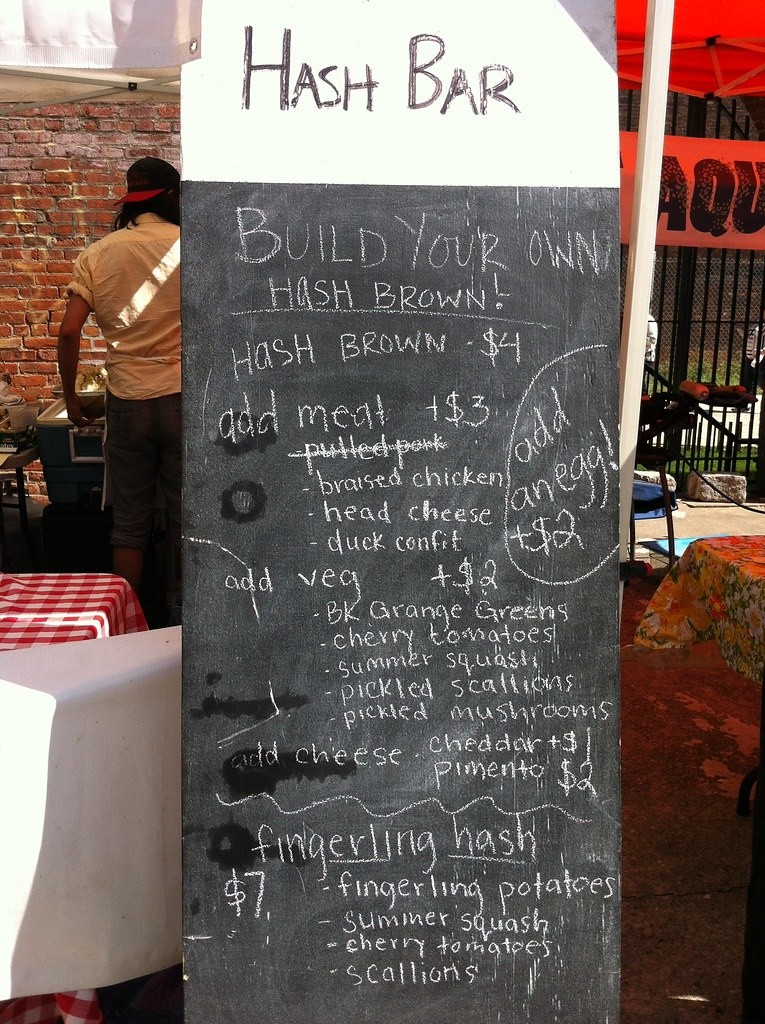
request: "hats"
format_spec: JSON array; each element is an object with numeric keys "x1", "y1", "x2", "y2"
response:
[{"x1": 113, "y1": 156, "x2": 181, "y2": 206}]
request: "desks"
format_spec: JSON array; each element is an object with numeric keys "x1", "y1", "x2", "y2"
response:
[
  {"x1": 0, "y1": 565, "x2": 150, "y2": 650},
  {"x1": 637, "y1": 533, "x2": 765, "y2": 814},
  {"x1": 0, "y1": 444, "x2": 39, "y2": 533}
]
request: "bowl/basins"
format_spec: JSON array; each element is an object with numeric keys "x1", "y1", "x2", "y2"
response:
[{"x1": 7, "y1": 406, "x2": 39, "y2": 428}]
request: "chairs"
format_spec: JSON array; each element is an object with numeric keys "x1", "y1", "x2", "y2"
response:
[{"x1": 626, "y1": 453, "x2": 677, "y2": 568}]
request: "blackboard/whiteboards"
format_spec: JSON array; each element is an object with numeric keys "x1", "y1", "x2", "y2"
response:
[{"x1": 180, "y1": 0, "x2": 622, "y2": 1024}]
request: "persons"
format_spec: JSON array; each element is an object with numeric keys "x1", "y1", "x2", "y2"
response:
[
  {"x1": 733, "y1": 305, "x2": 765, "y2": 413},
  {"x1": 57, "y1": 156, "x2": 181, "y2": 607},
  {"x1": 640, "y1": 312, "x2": 659, "y2": 394}
]
